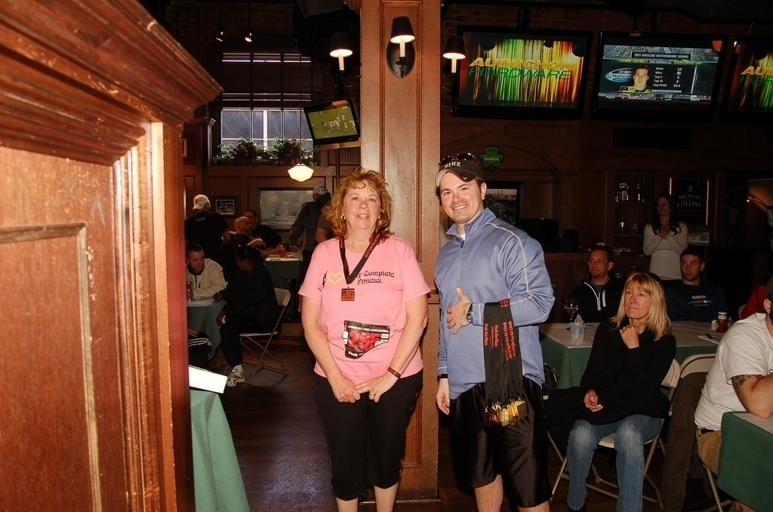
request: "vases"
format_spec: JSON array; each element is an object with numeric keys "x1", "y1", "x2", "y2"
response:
[
  {"x1": 276, "y1": 148, "x2": 300, "y2": 166},
  {"x1": 232, "y1": 154, "x2": 256, "y2": 166}
]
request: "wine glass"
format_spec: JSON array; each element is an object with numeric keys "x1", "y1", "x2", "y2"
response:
[
  {"x1": 617, "y1": 214, "x2": 640, "y2": 234},
  {"x1": 564, "y1": 297, "x2": 579, "y2": 330}
]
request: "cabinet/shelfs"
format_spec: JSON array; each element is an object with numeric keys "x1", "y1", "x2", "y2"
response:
[{"x1": 614, "y1": 198, "x2": 648, "y2": 239}]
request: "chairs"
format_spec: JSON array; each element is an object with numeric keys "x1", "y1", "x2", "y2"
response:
[
  {"x1": 180, "y1": 243, "x2": 300, "y2": 393},
  {"x1": 546, "y1": 353, "x2": 735, "y2": 509}
]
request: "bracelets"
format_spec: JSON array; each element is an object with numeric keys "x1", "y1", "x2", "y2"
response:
[{"x1": 388, "y1": 367, "x2": 401, "y2": 378}]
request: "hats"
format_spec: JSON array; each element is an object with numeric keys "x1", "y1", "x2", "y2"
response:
[
  {"x1": 313, "y1": 185, "x2": 329, "y2": 195},
  {"x1": 192, "y1": 194, "x2": 209, "y2": 210},
  {"x1": 434, "y1": 152, "x2": 483, "y2": 188}
]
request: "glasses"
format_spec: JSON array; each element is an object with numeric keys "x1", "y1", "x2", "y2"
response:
[{"x1": 438, "y1": 153, "x2": 477, "y2": 167}]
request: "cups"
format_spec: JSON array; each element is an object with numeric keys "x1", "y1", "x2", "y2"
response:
[{"x1": 568, "y1": 322, "x2": 586, "y2": 345}]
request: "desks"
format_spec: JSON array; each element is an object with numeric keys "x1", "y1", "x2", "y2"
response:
[
  {"x1": 185, "y1": 380, "x2": 253, "y2": 511},
  {"x1": 540, "y1": 319, "x2": 734, "y2": 389},
  {"x1": 715, "y1": 407, "x2": 772, "y2": 510}
]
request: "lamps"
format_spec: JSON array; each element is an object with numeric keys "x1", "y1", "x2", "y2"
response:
[
  {"x1": 439, "y1": 36, "x2": 467, "y2": 78},
  {"x1": 242, "y1": 31, "x2": 254, "y2": 44},
  {"x1": 287, "y1": 52, "x2": 315, "y2": 184},
  {"x1": 327, "y1": 32, "x2": 360, "y2": 82},
  {"x1": 383, "y1": 15, "x2": 417, "y2": 80},
  {"x1": 215, "y1": 30, "x2": 225, "y2": 42}
]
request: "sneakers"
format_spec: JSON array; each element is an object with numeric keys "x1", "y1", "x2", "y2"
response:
[{"x1": 225, "y1": 363, "x2": 247, "y2": 388}]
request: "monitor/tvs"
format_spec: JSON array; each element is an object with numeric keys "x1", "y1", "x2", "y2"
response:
[
  {"x1": 303, "y1": 98, "x2": 360, "y2": 145},
  {"x1": 451, "y1": 25, "x2": 593, "y2": 119},
  {"x1": 591, "y1": 29, "x2": 729, "y2": 123}
]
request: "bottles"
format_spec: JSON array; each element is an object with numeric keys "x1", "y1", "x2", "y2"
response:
[
  {"x1": 614, "y1": 178, "x2": 644, "y2": 203},
  {"x1": 279, "y1": 239, "x2": 286, "y2": 258},
  {"x1": 187, "y1": 281, "x2": 194, "y2": 300},
  {"x1": 712, "y1": 311, "x2": 732, "y2": 334}
]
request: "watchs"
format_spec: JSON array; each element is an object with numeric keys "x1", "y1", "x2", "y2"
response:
[{"x1": 466, "y1": 304, "x2": 473, "y2": 323}]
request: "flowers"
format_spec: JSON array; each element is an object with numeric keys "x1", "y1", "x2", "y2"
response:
[
  {"x1": 213, "y1": 135, "x2": 269, "y2": 165},
  {"x1": 268, "y1": 134, "x2": 303, "y2": 158}
]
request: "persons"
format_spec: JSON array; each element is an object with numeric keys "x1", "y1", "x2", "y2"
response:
[
  {"x1": 622, "y1": 65, "x2": 651, "y2": 93},
  {"x1": 185, "y1": 186, "x2": 337, "y2": 387},
  {"x1": 310, "y1": 111, "x2": 355, "y2": 131},
  {"x1": 298, "y1": 167, "x2": 432, "y2": 512},
  {"x1": 433, "y1": 150, "x2": 555, "y2": 512}
]
